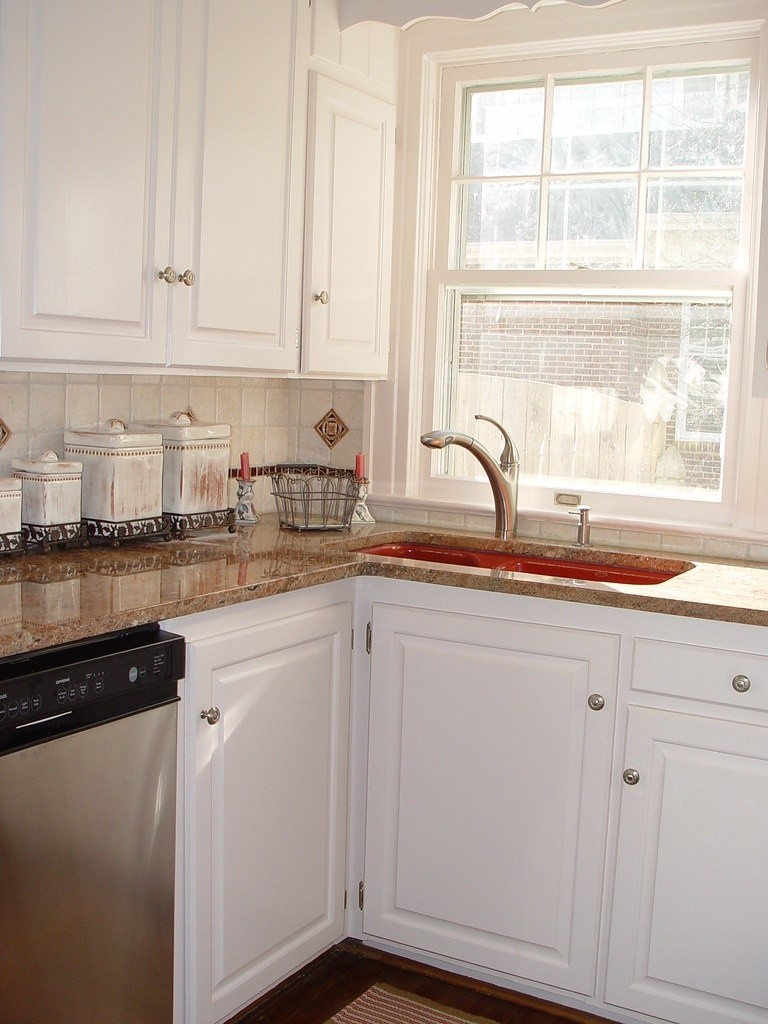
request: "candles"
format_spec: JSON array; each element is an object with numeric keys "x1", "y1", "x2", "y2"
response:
[
  {"x1": 241, "y1": 452, "x2": 250, "y2": 480},
  {"x1": 356, "y1": 452, "x2": 365, "y2": 478}
]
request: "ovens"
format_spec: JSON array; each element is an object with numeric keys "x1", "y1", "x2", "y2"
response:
[{"x1": 0, "y1": 623, "x2": 186, "y2": 1024}]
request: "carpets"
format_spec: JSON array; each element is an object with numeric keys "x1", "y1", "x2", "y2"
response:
[{"x1": 320, "y1": 981, "x2": 499, "y2": 1024}]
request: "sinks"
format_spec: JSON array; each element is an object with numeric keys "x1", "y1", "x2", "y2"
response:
[{"x1": 319, "y1": 530, "x2": 697, "y2": 587}]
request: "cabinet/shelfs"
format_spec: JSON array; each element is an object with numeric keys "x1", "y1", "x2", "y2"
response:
[
  {"x1": 1, "y1": 0, "x2": 398, "y2": 382},
  {"x1": 157, "y1": 576, "x2": 768, "y2": 1024}
]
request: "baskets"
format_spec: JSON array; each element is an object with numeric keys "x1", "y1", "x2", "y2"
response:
[{"x1": 270, "y1": 468, "x2": 362, "y2": 532}]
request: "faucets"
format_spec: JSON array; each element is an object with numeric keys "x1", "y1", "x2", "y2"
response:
[{"x1": 420, "y1": 414, "x2": 520, "y2": 540}]
